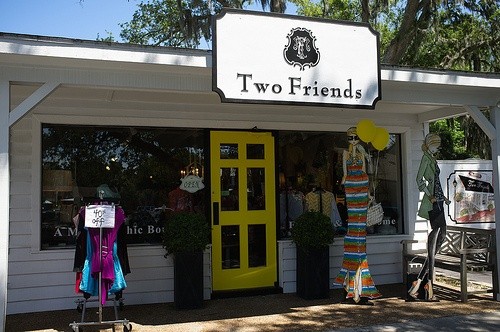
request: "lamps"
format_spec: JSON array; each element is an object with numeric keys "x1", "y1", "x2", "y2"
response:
[{"x1": 180, "y1": 133, "x2": 205, "y2": 184}]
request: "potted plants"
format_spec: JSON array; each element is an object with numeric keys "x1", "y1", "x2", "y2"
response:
[
  {"x1": 290, "y1": 211, "x2": 337, "y2": 300},
  {"x1": 162, "y1": 212, "x2": 212, "y2": 309}
]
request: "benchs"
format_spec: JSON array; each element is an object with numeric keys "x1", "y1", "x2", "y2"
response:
[{"x1": 400, "y1": 225, "x2": 496, "y2": 303}]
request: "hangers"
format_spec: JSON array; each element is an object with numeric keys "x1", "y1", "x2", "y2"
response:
[
  {"x1": 280, "y1": 179, "x2": 327, "y2": 196},
  {"x1": 75, "y1": 190, "x2": 121, "y2": 209}
]
